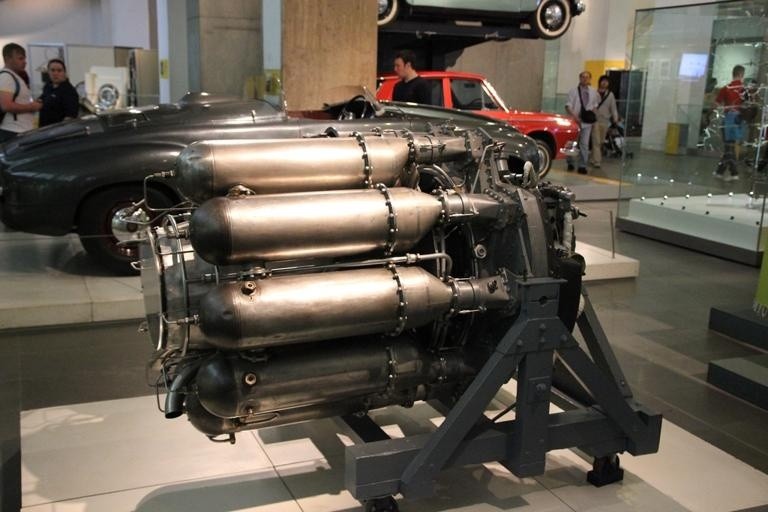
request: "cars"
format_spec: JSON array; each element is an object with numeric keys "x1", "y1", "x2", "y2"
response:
[
  {"x1": 374, "y1": 70, "x2": 582, "y2": 179},
  {"x1": 378, "y1": 0, "x2": 586, "y2": 52}
]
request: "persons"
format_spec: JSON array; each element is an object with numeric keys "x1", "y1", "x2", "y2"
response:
[
  {"x1": 38, "y1": 59, "x2": 79, "y2": 128},
  {"x1": 0, "y1": 43, "x2": 43, "y2": 143},
  {"x1": 703, "y1": 64, "x2": 759, "y2": 182},
  {"x1": 589, "y1": 75, "x2": 618, "y2": 168},
  {"x1": 565, "y1": 71, "x2": 597, "y2": 174},
  {"x1": 392, "y1": 49, "x2": 432, "y2": 104}
]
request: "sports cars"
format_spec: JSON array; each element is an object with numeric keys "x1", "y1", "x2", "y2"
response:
[{"x1": 0, "y1": 82, "x2": 540, "y2": 271}]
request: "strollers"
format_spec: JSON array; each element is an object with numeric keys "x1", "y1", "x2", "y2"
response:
[{"x1": 602, "y1": 117, "x2": 633, "y2": 159}]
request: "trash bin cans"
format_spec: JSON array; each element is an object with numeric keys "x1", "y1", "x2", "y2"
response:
[{"x1": 664, "y1": 123, "x2": 690, "y2": 156}]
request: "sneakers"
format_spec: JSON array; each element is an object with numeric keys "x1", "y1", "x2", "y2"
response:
[
  {"x1": 712, "y1": 171, "x2": 739, "y2": 182},
  {"x1": 568, "y1": 164, "x2": 575, "y2": 172},
  {"x1": 594, "y1": 163, "x2": 600, "y2": 167},
  {"x1": 577, "y1": 167, "x2": 587, "y2": 174}
]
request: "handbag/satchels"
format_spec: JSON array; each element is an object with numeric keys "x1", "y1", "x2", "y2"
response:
[{"x1": 580, "y1": 106, "x2": 597, "y2": 124}]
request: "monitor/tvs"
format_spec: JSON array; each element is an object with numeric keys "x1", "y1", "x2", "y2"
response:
[{"x1": 678, "y1": 53, "x2": 707, "y2": 79}]
